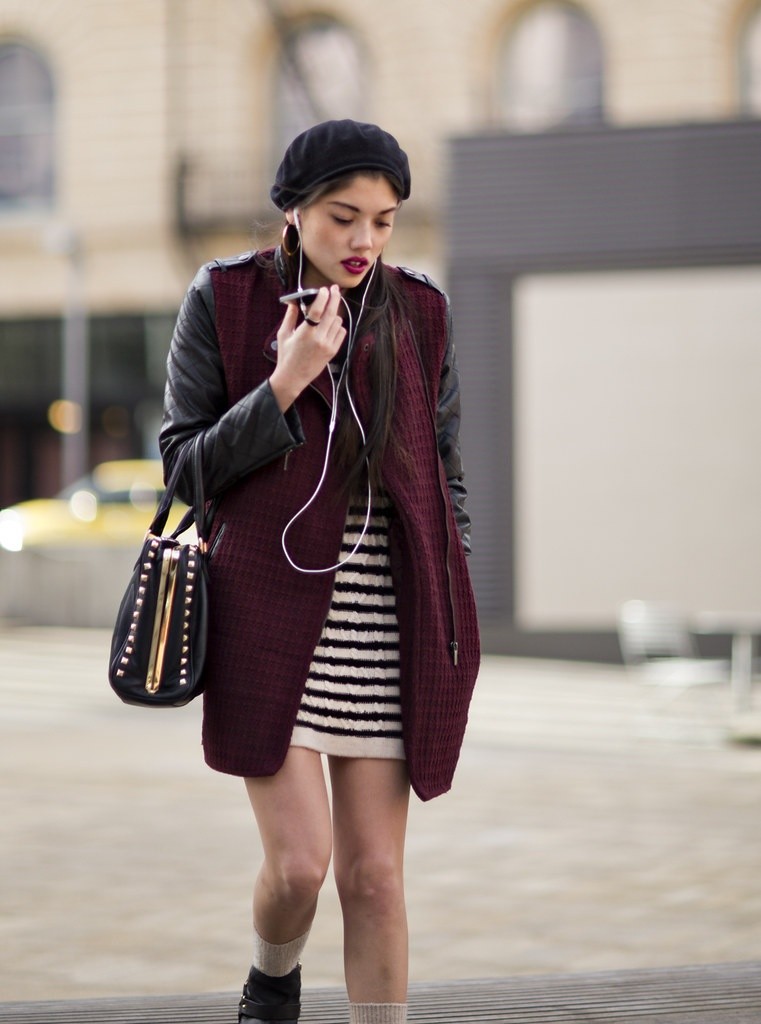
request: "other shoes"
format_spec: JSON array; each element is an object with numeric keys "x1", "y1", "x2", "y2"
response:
[{"x1": 239, "y1": 965, "x2": 301, "y2": 1024}]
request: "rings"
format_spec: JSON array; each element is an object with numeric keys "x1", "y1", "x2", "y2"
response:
[{"x1": 304, "y1": 316, "x2": 321, "y2": 326}]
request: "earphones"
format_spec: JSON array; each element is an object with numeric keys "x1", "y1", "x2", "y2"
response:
[{"x1": 293, "y1": 206, "x2": 301, "y2": 231}]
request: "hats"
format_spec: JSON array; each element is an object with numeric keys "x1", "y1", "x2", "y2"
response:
[{"x1": 270, "y1": 119, "x2": 410, "y2": 210}]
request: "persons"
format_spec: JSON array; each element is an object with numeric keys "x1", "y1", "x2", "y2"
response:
[{"x1": 157, "y1": 120, "x2": 481, "y2": 1024}]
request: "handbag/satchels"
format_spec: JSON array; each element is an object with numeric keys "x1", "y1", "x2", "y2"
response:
[{"x1": 108, "y1": 429, "x2": 234, "y2": 708}]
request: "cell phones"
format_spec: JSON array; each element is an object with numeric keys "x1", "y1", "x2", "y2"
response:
[{"x1": 280, "y1": 289, "x2": 319, "y2": 310}]
